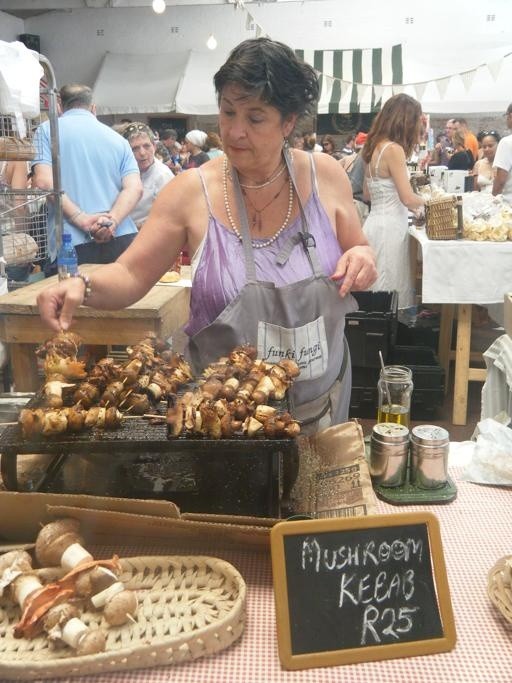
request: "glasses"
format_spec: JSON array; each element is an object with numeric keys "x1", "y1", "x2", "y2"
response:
[{"x1": 205, "y1": 31, "x2": 217, "y2": 49}]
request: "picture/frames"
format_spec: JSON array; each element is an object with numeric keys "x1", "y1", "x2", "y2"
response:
[
  {"x1": 0, "y1": 258, "x2": 193, "y2": 390},
  {"x1": 409, "y1": 216, "x2": 512, "y2": 433}
]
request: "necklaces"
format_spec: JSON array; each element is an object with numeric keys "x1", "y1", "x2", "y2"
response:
[{"x1": 223, "y1": 154, "x2": 294, "y2": 248}]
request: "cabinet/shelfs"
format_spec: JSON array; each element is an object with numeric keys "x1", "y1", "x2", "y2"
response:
[
  {"x1": 369, "y1": 421, "x2": 409, "y2": 488},
  {"x1": 55, "y1": 233, "x2": 78, "y2": 283},
  {"x1": 411, "y1": 425, "x2": 450, "y2": 491}
]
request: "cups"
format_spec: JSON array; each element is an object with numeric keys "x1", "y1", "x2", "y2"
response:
[
  {"x1": 482, "y1": 130, "x2": 497, "y2": 136},
  {"x1": 322, "y1": 142, "x2": 329, "y2": 147},
  {"x1": 123, "y1": 124, "x2": 150, "y2": 138},
  {"x1": 502, "y1": 113, "x2": 509, "y2": 118}
]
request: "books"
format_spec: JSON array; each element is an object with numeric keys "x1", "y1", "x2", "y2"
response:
[
  {"x1": 345, "y1": 366, "x2": 385, "y2": 419},
  {"x1": 345, "y1": 289, "x2": 400, "y2": 371},
  {"x1": 391, "y1": 343, "x2": 445, "y2": 422}
]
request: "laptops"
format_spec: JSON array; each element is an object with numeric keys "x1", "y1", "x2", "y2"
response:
[
  {"x1": 1, "y1": 136, "x2": 37, "y2": 160},
  {"x1": 3, "y1": 233, "x2": 39, "y2": 264}
]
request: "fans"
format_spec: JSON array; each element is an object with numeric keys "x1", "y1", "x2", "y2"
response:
[{"x1": 270, "y1": 511, "x2": 456, "y2": 672}]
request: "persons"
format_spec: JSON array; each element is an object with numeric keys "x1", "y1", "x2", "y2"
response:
[
  {"x1": 29, "y1": 83, "x2": 143, "y2": 277},
  {"x1": 1, "y1": 159, "x2": 47, "y2": 281},
  {"x1": 289, "y1": 129, "x2": 368, "y2": 204},
  {"x1": 36, "y1": 38, "x2": 379, "y2": 436},
  {"x1": 418, "y1": 117, "x2": 485, "y2": 192},
  {"x1": 152, "y1": 129, "x2": 224, "y2": 174},
  {"x1": 473, "y1": 132, "x2": 501, "y2": 192},
  {"x1": 362, "y1": 93, "x2": 427, "y2": 309},
  {"x1": 492, "y1": 107, "x2": 512, "y2": 197},
  {"x1": 120, "y1": 122, "x2": 176, "y2": 231}
]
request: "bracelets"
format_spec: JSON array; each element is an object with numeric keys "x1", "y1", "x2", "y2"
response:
[{"x1": 59, "y1": 273, "x2": 91, "y2": 304}]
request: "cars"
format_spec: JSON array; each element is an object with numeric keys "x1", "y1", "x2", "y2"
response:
[
  {"x1": 168, "y1": 251, "x2": 184, "y2": 274},
  {"x1": 376, "y1": 364, "x2": 414, "y2": 429}
]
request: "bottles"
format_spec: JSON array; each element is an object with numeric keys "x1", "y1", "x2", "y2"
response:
[{"x1": 355, "y1": 131, "x2": 367, "y2": 149}]
